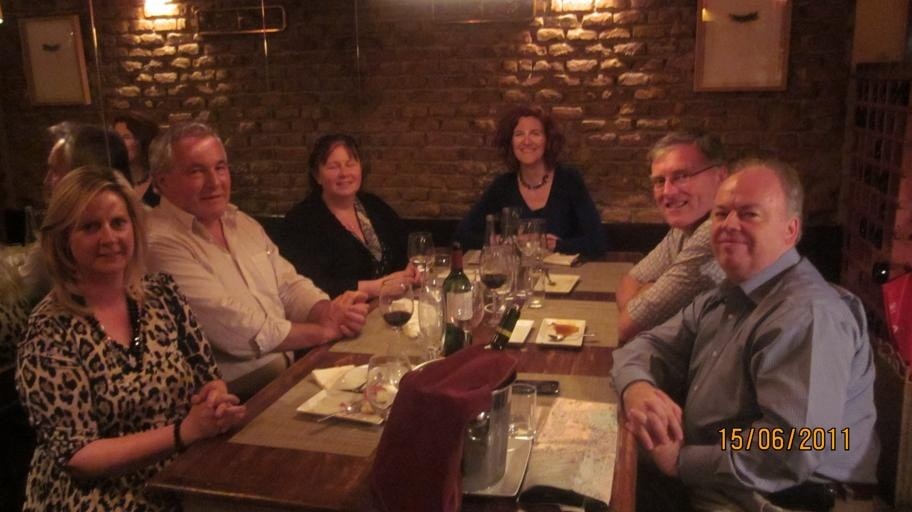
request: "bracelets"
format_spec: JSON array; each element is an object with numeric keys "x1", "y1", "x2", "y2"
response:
[{"x1": 173, "y1": 418, "x2": 185, "y2": 455}]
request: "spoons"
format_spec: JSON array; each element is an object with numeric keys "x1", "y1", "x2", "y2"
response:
[
  {"x1": 315, "y1": 401, "x2": 363, "y2": 421},
  {"x1": 545, "y1": 273, "x2": 556, "y2": 289},
  {"x1": 549, "y1": 328, "x2": 579, "y2": 342}
]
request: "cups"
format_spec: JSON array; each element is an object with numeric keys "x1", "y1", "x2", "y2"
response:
[
  {"x1": 449, "y1": 282, "x2": 485, "y2": 353},
  {"x1": 514, "y1": 220, "x2": 545, "y2": 297},
  {"x1": 408, "y1": 231, "x2": 433, "y2": 296},
  {"x1": 507, "y1": 384, "x2": 538, "y2": 439},
  {"x1": 419, "y1": 286, "x2": 445, "y2": 360},
  {"x1": 365, "y1": 353, "x2": 413, "y2": 431},
  {"x1": 431, "y1": 252, "x2": 451, "y2": 284},
  {"x1": 378, "y1": 279, "x2": 414, "y2": 352},
  {"x1": 479, "y1": 244, "x2": 508, "y2": 325}
]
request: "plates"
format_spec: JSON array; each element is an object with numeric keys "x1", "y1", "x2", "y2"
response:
[
  {"x1": 535, "y1": 318, "x2": 586, "y2": 347},
  {"x1": 532, "y1": 273, "x2": 581, "y2": 294},
  {"x1": 342, "y1": 364, "x2": 418, "y2": 393},
  {"x1": 462, "y1": 437, "x2": 535, "y2": 498},
  {"x1": 296, "y1": 387, "x2": 394, "y2": 426}
]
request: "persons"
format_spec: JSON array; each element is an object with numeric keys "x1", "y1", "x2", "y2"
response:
[
  {"x1": 16, "y1": 167, "x2": 246, "y2": 511},
  {"x1": 460, "y1": 107, "x2": 606, "y2": 262},
  {"x1": 19, "y1": 121, "x2": 129, "y2": 293},
  {"x1": 113, "y1": 115, "x2": 163, "y2": 207},
  {"x1": 611, "y1": 159, "x2": 890, "y2": 510},
  {"x1": 143, "y1": 124, "x2": 370, "y2": 403},
  {"x1": 276, "y1": 136, "x2": 427, "y2": 297},
  {"x1": 614, "y1": 128, "x2": 734, "y2": 345}
]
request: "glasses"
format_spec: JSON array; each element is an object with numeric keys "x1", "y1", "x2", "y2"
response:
[{"x1": 650, "y1": 163, "x2": 718, "y2": 183}]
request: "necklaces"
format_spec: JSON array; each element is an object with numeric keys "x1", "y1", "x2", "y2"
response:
[
  {"x1": 516, "y1": 170, "x2": 547, "y2": 189},
  {"x1": 58, "y1": 289, "x2": 146, "y2": 373}
]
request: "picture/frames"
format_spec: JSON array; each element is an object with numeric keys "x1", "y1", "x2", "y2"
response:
[
  {"x1": 18, "y1": 12, "x2": 92, "y2": 102},
  {"x1": 689, "y1": 1, "x2": 796, "y2": 94}
]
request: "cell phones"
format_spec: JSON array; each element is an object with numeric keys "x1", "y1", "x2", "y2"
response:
[{"x1": 512, "y1": 379, "x2": 559, "y2": 397}]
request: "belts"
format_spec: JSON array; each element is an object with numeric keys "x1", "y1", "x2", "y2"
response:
[{"x1": 806, "y1": 480, "x2": 880, "y2": 500}]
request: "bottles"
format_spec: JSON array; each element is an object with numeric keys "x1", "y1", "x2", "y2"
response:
[
  {"x1": 479, "y1": 306, "x2": 521, "y2": 386},
  {"x1": 871, "y1": 261, "x2": 911, "y2": 283},
  {"x1": 498, "y1": 210, "x2": 516, "y2": 291},
  {"x1": 442, "y1": 242, "x2": 472, "y2": 355}
]
